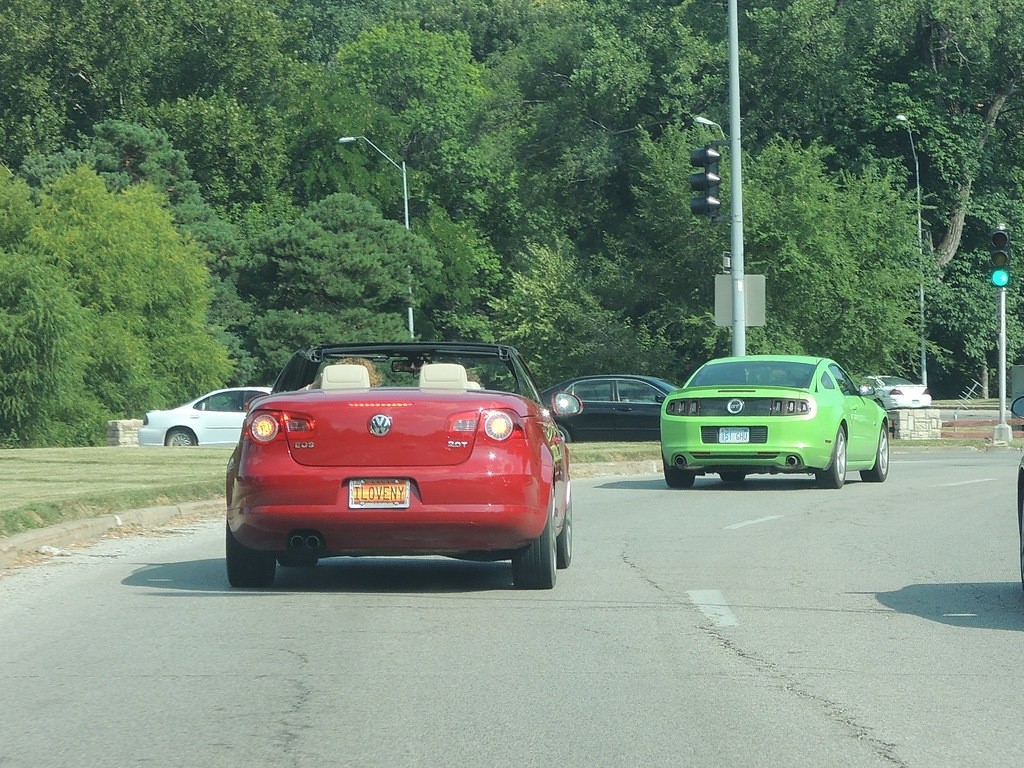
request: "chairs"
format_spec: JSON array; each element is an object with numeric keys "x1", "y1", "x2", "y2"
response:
[
  {"x1": 418, "y1": 364, "x2": 467, "y2": 389},
  {"x1": 721, "y1": 366, "x2": 746, "y2": 383},
  {"x1": 320, "y1": 365, "x2": 370, "y2": 388}
]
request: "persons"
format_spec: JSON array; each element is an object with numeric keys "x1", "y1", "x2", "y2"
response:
[
  {"x1": 465, "y1": 365, "x2": 482, "y2": 382},
  {"x1": 291, "y1": 357, "x2": 383, "y2": 391}
]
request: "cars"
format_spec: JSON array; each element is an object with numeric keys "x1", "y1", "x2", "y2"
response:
[
  {"x1": 137, "y1": 385, "x2": 272, "y2": 446},
  {"x1": 224, "y1": 340, "x2": 583, "y2": 588},
  {"x1": 661, "y1": 353, "x2": 888, "y2": 489},
  {"x1": 860, "y1": 377, "x2": 932, "y2": 410},
  {"x1": 537, "y1": 374, "x2": 681, "y2": 439}
]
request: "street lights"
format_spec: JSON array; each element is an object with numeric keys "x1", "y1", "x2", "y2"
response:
[
  {"x1": 340, "y1": 136, "x2": 415, "y2": 341},
  {"x1": 899, "y1": 115, "x2": 929, "y2": 384},
  {"x1": 695, "y1": 115, "x2": 726, "y2": 140}
]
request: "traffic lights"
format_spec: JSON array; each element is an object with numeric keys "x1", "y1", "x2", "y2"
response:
[
  {"x1": 687, "y1": 144, "x2": 722, "y2": 216},
  {"x1": 991, "y1": 231, "x2": 1012, "y2": 287}
]
